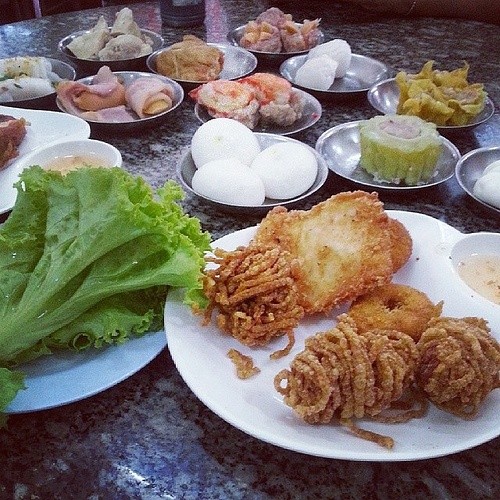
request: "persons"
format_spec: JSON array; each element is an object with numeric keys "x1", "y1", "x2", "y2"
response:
[{"x1": 357, "y1": 0, "x2": 500, "y2": 25}]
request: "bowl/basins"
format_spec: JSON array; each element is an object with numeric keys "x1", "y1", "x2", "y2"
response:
[
  {"x1": 177, "y1": 132, "x2": 329, "y2": 212},
  {"x1": 57, "y1": 70, "x2": 185, "y2": 131},
  {"x1": 193, "y1": 89, "x2": 323, "y2": 137},
  {"x1": 59, "y1": 26, "x2": 165, "y2": 70},
  {"x1": 455, "y1": 145, "x2": 500, "y2": 214},
  {"x1": 367, "y1": 79, "x2": 495, "y2": 135},
  {"x1": 279, "y1": 54, "x2": 388, "y2": 98},
  {"x1": 146, "y1": 42, "x2": 258, "y2": 89},
  {"x1": 0, "y1": 57, "x2": 76, "y2": 106},
  {"x1": 226, "y1": 22, "x2": 325, "y2": 64},
  {"x1": 317, "y1": 120, "x2": 461, "y2": 192}
]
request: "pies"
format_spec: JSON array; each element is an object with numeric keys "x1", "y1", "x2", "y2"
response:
[
  {"x1": 0, "y1": 55, "x2": 60, "y2": 102},
  {"x1": 64, "y1": 8, "x2": 152, "y2": 60},
  {"x1": 156, "y1": 34, "x2": 225, "y2": 81}
]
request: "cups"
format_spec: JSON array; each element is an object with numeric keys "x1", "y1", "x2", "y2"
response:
[{"x1": 160, "y1": 0, "x2": 206, "y2": 28}]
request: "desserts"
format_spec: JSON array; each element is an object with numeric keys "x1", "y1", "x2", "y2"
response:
[
  {"x1": 188, "y1": 70, "x2": 306, "y2": 129},
  {"x1": 359, "y1": 114, "x2": 442, "y2": 186},
  {"x1": 396, "y1": 58, "x2": 489, "y2": 126}
]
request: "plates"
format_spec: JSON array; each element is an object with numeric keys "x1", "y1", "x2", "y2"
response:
[
  {"x1": 163, "y1": 211, "x2": 500, "y2": 462},
  {"x1": 0, "y1": 327, "x2": 167, "y2": 414},
  {"x1": 0, "y1": 104, "x2": 122, "y2": 216}
]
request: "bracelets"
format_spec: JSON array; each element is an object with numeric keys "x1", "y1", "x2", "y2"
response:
[{"x1": 403, "y1": 0, "x2": 417, "y2": 16}]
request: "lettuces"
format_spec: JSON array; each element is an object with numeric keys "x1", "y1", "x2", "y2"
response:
[{"x1": 0, "y1": 164, "x2": 213, "y2": 412}]
